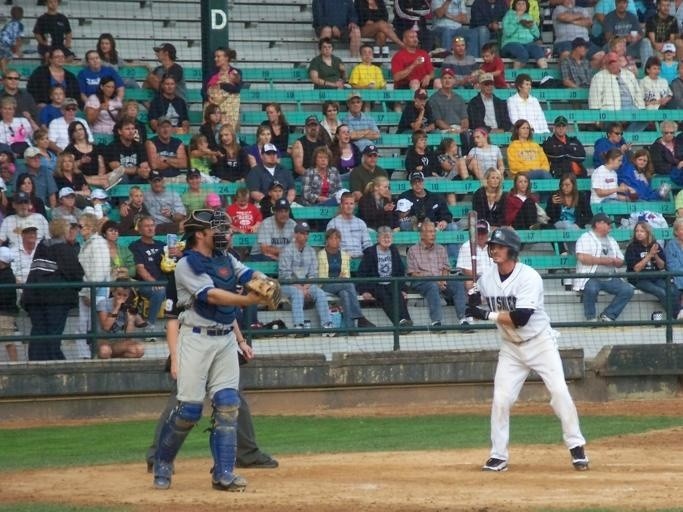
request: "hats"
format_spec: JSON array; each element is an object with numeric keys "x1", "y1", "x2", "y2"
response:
[
  {"x1": 5, "y1": 67, "x2": 311, "y2": 232},
  {"x1": 555, "y1": 37, "x2": 676, "y2": 223},
  {"x1": 318, "y1": 69, "x2": 494, "y2": 180}
]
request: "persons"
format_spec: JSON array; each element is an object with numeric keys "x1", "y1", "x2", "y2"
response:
[
  {"x1": 153, "y1": 211, "x2": 271, "y2": 493},
  {"x1": 0, "y1": 0, "x2": 683, "y2": 359},
  {"x1": 465, "y1": 232, "x2": 588, "y2": 471},
  {"x1": 147, "y1": 223, "x2": 279, "y2": 470}
]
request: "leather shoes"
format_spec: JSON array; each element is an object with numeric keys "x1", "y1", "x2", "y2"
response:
[{"x1": 134, "y1": 318, "x2": 147, "y2": 328}]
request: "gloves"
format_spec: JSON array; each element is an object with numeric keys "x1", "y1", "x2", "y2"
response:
[{"x1": 464, "y1": 303, "x2": 490, "y2": 320}]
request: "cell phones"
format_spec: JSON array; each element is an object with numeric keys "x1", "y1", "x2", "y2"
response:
[{"x1": 554, "y1": 190, "x2": 561, "y2": 204}]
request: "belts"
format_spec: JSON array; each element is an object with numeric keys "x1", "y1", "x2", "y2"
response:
[{"x1": 193, "y1": 326, "x2": 233, "y2": 337}]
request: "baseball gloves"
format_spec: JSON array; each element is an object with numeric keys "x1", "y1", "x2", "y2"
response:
[{"x1": 246, "y1": 277, "x2": 282, "y2": 309}]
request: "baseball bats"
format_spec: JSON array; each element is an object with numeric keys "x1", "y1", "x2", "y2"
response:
[{"x1": 468, "y1": 210, "x2": 477, "y2": 289}]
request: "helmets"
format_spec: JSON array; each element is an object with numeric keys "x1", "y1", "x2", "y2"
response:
[
  {"x1": 178, "y1": 208, "x2": 234, "y2": 257},
  {"x1": 484, "y1": 227, "x2": 522, "y2": 261}
]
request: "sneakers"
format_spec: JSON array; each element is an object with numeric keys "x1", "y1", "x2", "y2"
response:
[
  {"x1": 569, "y1": 446, "x2": 589, "y2": 465},
  {"x1": 600, "y1": 313, "x2": 613, "y2": 322},
  {"x1": 211, "y1": 471, "x2": 247, "y2": 492},
  {"x1": 235, "y1": 452, "x2": 279, "y2": 468},
  {"x1": 321, "y1": 325, "x2": 337, "y2": 337},
  {"x1": 144, "y1": 325, "x2": 158, "y2": 342},
  {"x1": 358, "y1": 318, "x2": 376, "y2": 327},
  {"x1": 460, "y1": 318, "x2": 474, "y2": 325},
  {"x1": 482, "y1": 457, "x2": 508, "y2": 471},
  {"x1": 152, "y1": 450, "x2": 172, "y2": 490}
]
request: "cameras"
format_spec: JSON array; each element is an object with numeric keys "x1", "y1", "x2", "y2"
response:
[
  {"x1": 419, "y1": 57, "x2": 424, "y2": 62},
  {"x1": 121, "y1": 303, "x2": 138, "y2": 315}
]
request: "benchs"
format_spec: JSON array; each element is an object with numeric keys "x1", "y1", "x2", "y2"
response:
[{"x1": 0, "y1": 1, "x2": 683, "y2": 305}]
